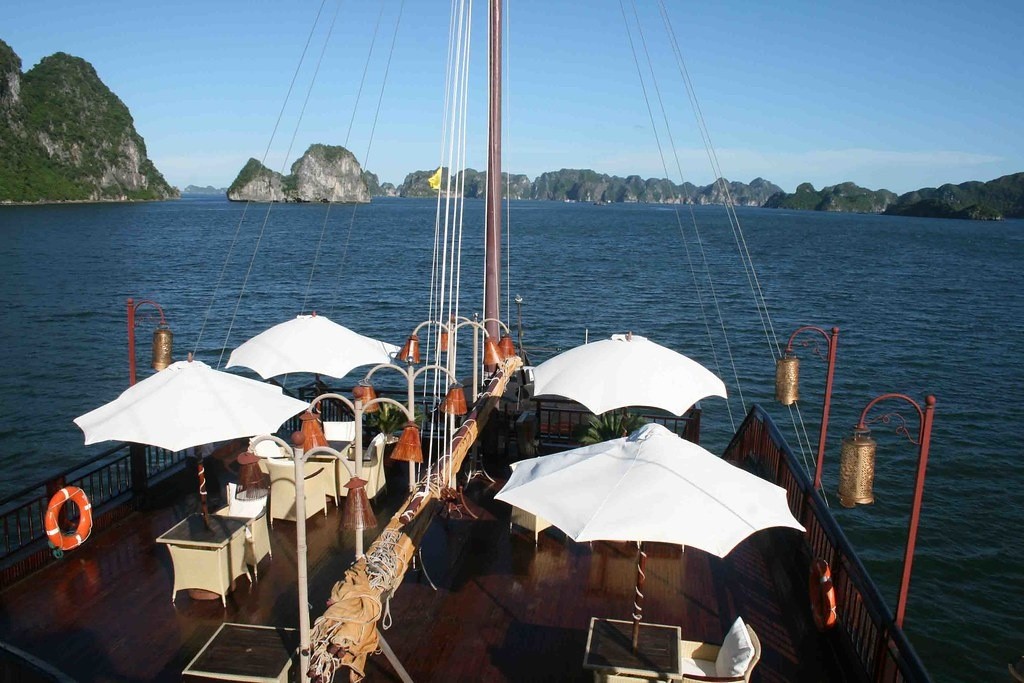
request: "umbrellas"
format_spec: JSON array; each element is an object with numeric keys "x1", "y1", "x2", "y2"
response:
[
  {"x1": 494, "y1": 423, "x2": 809, "y2": 657},
  {"x1": 224, "y1": 307, "x2": 403, "y2": 432},
  {"x1": 71, "y1": 350, "x2": 312, "y2": 529},
  {"x1": 531, "y1": 330, "x2": 729, "y2": 441}
]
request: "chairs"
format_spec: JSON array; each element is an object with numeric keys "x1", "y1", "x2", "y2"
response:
[
  {"x1": 509, "y1": 502, "x2": 554, "y2": 546},
  {"x1": 335, "y1": 432, "x2": 389, "y2": 506},
  {"x1": 248, "y1": 432, "x2": 294, "y2": 475},
  {"x1": 211, "y1": 483, "x2": 273, "y2": 578},
  {"x1": 266, "y1": 459, "x2": 328, "y2": 526},
  {"x1": 671, "y1": 618, "x2": 762, "y2": 683}
]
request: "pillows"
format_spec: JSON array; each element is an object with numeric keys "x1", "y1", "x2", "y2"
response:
[
  {"x1": 249, "y1": 434, "x2": 281, "y2": 461},
  {"x1": 268, "y1": 459, "x2": 303, "y2": 468},
  {"x1": 225, "y1": 482, "x2": 268, "y2": 520},
  {"x1": 363, "y1": 432, "x2": 387, "y2": 461},
  {"x1": 321, "y1": 421, "x2": 357, "y2": 441},
  {"x1": 715, "y1": 615, "x2": 755, "y2": 682}
]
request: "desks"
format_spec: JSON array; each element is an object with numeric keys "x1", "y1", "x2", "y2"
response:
[
  {"x1": 582, "y1": 617, "x2": 684, "y2": 683},
  {"x1": 156, "y1": 512, "x2": 257, "y2": 609},
  {"x1": 182, "y1": 620, "x2": 313, "y2": 682},
  {"x1": 298, "y1": 440, "x2": 353, "y2": 501}
]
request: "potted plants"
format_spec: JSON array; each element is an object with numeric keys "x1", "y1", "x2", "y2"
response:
[{"x1": 366, "y1": 398, "x2": 428, "y2": 467}]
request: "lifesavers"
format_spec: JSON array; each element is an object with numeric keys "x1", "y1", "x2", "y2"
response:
[
  {"x1": 809, "y1": 558, "x2": 837, "y2": 632},
  {"x1": 44, "y1": 486, "x2": 93, "y2": 551}
]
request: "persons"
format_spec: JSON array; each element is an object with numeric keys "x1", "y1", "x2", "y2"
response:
[{"x1": 185, "y1": 439, "x2": 221, "y2": 494}]
